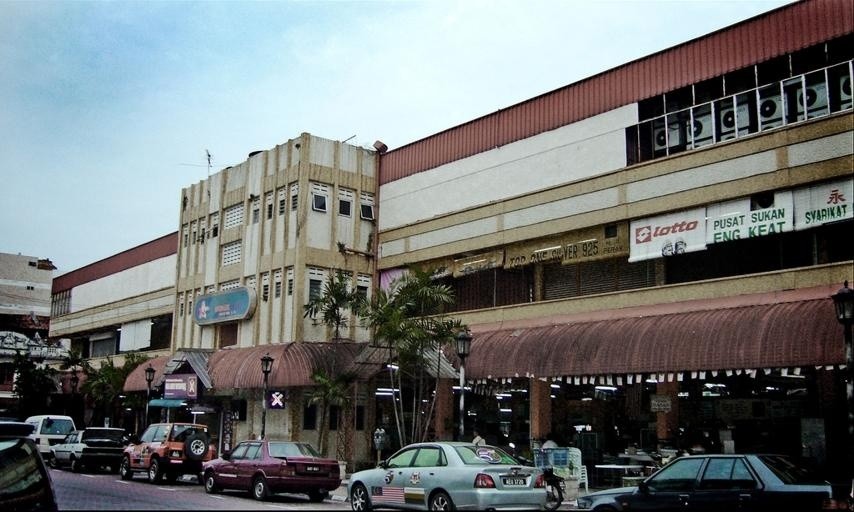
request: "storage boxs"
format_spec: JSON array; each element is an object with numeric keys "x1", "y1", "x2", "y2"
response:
[{"x1": 533, "y1": 448, "x2": 568, "y2": 467}]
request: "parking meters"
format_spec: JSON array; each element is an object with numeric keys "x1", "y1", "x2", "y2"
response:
[{"x1": 374, "y1": 428, "x2": 386, "y2": 468}]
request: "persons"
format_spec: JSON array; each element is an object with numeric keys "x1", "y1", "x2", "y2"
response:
[{"x1": 472, "y1": 427, "x2": 485, "y2": 445}]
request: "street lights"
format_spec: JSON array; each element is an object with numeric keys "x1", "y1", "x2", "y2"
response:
[
  {"x1": 70, "y1": 369, "x2": 80, "y2": 417},
  {"x1": 453, "y1": 323, "x2": 471, "y2": 438},
  {"x1": 144, "y1": 363, "x2": 156, "y2": 428},
  {"x1": 829, "y1": 279, "x2": 854, "y2": 511},
  {"x1": 260, "y1": 354, "x2": 273, "y2": 439}
]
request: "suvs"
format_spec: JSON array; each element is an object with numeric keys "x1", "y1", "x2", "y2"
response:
[{"x1": 120, "y1": 422, "x2": 215, "y2": 484}]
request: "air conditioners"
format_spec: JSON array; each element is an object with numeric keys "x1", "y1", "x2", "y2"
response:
[{"x1": 653, "y1": 75, "x2": 852, "y2": 152}]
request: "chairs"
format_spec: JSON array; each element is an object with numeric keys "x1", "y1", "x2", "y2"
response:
[{"x1": 568, "y1": 447, "x2": 588, "y2": 494}]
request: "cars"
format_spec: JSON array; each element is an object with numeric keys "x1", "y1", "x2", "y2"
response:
[
  {"x1": 50, "y1": 426, "x2": 131, "y2": 473},
  {"x1": 201, "y1": 439, "x2": 341, "y2": 501},
  {"x1": 347, "y1": 441, "x2": 547, "y2": 511},
  {"x1": 0, "y1": 419, "x2": 58, "y2": 511},
  {"x1": 578, "y1": 451, "x2": 835, "y2": 511}
]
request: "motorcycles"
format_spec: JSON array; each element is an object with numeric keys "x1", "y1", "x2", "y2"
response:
[{"x1": 509, "y1": 442, "x2": 566, "y2": 511}]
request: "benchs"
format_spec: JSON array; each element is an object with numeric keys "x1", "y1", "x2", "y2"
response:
[{"x1": 595, "y1": 464, "x2": 656, "y2": 487}]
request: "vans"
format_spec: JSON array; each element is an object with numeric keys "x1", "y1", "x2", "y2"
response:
[{"x1": 25, "y1": 414, "x2": 76, "y2": 455}]
